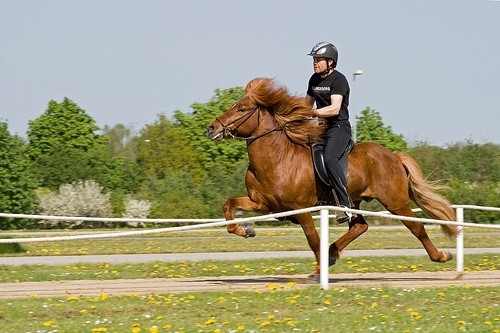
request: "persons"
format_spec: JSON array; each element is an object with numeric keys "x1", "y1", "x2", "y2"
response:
[{"x1": 304, "y1": 41, "x2": 359, "y2": 224}]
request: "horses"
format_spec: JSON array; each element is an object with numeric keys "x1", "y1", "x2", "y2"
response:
[{"x1": 207, "y1": 77, "x2": 464, "y2": 277}]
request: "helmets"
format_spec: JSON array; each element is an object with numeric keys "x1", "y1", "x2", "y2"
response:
[{"x1": 306, "y1": 42, "x2": 337, "y2": 75}]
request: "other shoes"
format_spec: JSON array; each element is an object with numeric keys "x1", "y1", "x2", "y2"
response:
[{"x1": 337, "y1": 209, "x2": 357, "y2": 223}]
